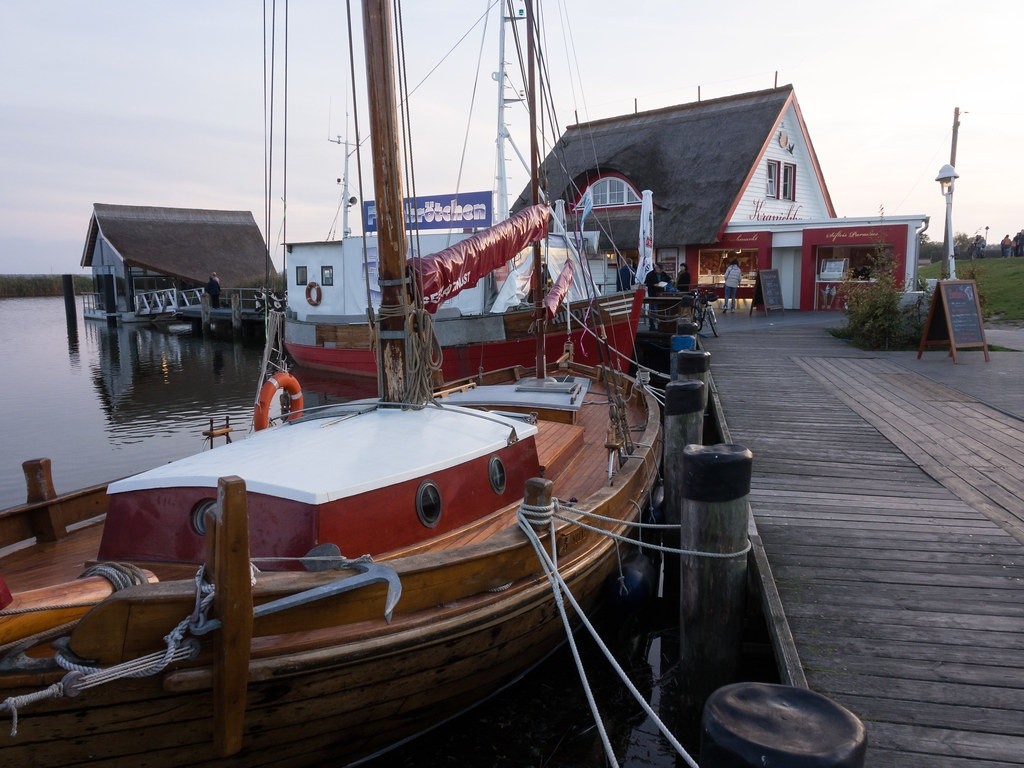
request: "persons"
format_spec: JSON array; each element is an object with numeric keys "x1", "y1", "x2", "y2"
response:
[
  {"x1": 643, "y1": 262, "x2": 674, "y2": 332},
  {"x1": 1000, "y1": 228, "x2": 1024, "y2": 258},
  {"x1": 722, "y1": 259, "x2": 741, "y2": 314},
  {"x1": 540, "y1": 263, "x2": 551, "y2": 287},
  {"x1": 616, "y1": 258, "x2": 636, "y2": 292},
  {"x1": 973, "y1": 235, "x2": 982, "y2": 258},
  {"x1": 674, "y1": 263, "x2": 691, "y2": 292},
  {"x1": 205, "y1": 272, "x2": 221, "y2": 309}
]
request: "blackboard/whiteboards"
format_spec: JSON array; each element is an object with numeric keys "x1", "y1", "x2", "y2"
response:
[
  {"x1": 923, "y1": 279, "x2": 985, "y2": 346},
  {"x1": 752, "y1": 269, "x2": 784, "y2": 308}
]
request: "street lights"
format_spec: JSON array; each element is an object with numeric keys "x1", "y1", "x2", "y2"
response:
[
  {"x1": 984, "y1": 226, "x2": 989, "y2": 254},
  {"x1": 935, "y1": 164, "x2": 960, "y2": 279}
]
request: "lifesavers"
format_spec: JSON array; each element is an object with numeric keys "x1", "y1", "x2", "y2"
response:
[
  {"x1": 305, "y1": 281, "x2": 322, "y2": 306},
  {"x1": 253, "y1": 372, "x2": 304, "y2": 432}
]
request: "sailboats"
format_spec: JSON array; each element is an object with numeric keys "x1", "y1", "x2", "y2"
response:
[
  {"x1": 280, "y1": 0, "x2": 647, "y2": 389},
  {"x1": 0, "y1": 0, "x2": 664, "y2": 768}
]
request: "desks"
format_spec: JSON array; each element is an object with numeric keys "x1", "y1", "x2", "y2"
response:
[{"x1": 644, "y1": 297, "x2": 695, "y2": 331}]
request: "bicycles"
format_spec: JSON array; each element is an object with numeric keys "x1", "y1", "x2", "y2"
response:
[
  {"x1": 971, "y1": 242, "x2": 986, "y2": 260},
  {"x1": 692, "y1": 288, "x2": 719, "y2": 338}
]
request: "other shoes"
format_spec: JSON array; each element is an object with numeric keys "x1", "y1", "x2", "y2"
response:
[
  {"x1": 731, "y1": 308, "x2": 735, "y2": 312},
  {"x1": 722, "y1": 306, "x2": 728, "y2": 313}
]
